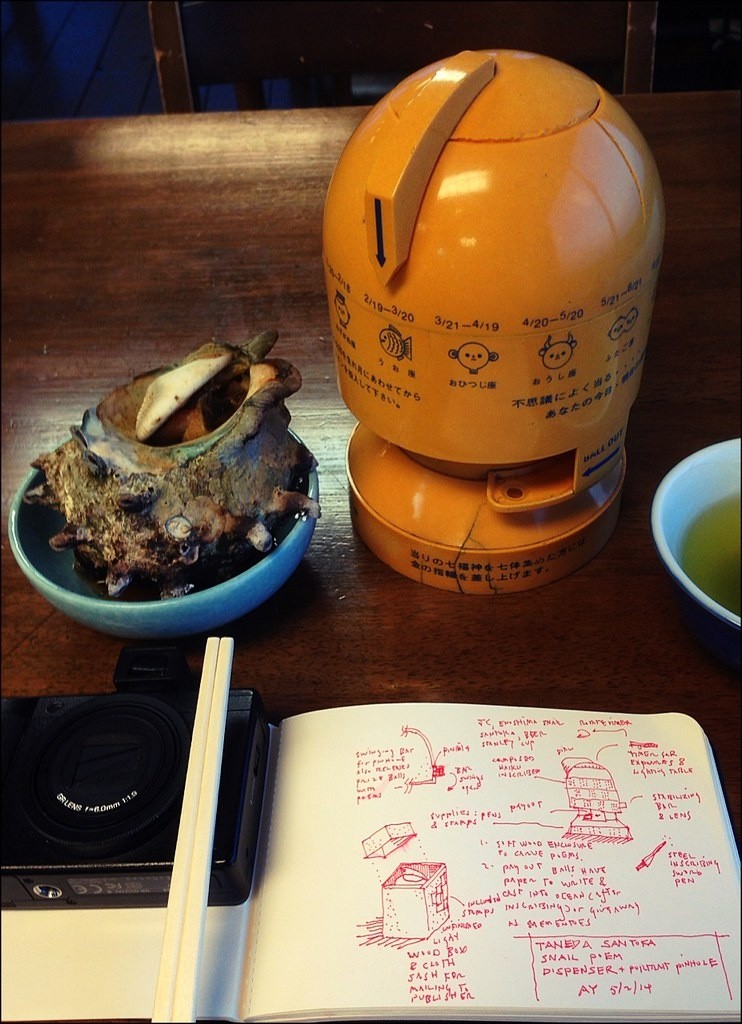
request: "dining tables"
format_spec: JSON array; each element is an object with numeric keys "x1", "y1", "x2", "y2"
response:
[{"x1": 1, "y1": 85, "x2": 742, "y2": 858}]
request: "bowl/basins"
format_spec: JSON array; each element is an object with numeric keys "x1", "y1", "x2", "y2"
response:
[
  {"x1": 652, "y1": 438, "x2": 742, "y2": 668},
  {"x1": 8, "y1": 427, "x2": 319, "y2": 638}
]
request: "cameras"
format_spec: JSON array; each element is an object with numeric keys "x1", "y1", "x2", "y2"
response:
[{"x1": 0, "y1": 634, "x2": 270, "y2": 910}]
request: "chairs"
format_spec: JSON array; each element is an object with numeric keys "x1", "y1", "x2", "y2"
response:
[{"x1": 146, "y1": 0, "x2": 658, "y2": 116}]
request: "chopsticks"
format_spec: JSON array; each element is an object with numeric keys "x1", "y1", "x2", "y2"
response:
[{"x1": 152, "y1": 636, "x2": 234, "y2": 1023}]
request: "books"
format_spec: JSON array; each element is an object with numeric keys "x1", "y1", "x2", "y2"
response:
[{"x1": 1, "y1": 703, "x2": 741, "y2": 1023}]
